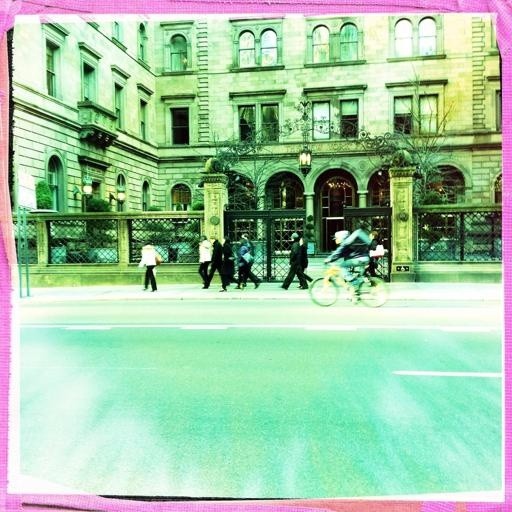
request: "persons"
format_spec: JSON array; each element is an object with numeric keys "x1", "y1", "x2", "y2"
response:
[
  {"x1": 278, "y1": 229, "x2": 381, "y2": 304},
  {"x1": 141, "y1": 243, "x2": 161, "y2": 292},
  {"x1": 197, "y1": 233, "x2": 261, "y2": 292}
]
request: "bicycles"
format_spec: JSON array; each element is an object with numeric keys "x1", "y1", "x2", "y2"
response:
[{"x1": 308, "y1": 260, "x2": 390, "y2": 309}]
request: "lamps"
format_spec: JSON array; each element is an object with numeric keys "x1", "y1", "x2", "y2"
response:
[
  {"x1": 299, "y1": 145, "x2": 312, "y2": 168},
  {"x1": 117, "y1": 187, "x2": 125, "y2": 201},
  {"x1": 83, "y1": 177, "x2": 92, "y2": 193}
]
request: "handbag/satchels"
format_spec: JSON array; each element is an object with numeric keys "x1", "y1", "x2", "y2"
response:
[
  {"x1": 155, "y1": 256, "x2": 161, "y2": 265},
  {"x1": 242, "y1": 253, "x2": 253, "y2": 263}
]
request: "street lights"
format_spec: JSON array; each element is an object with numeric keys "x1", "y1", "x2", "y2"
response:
[
  {"x1": 117, "y1": 191, "x2": 126, "y2": 213},
  {"x1": 83, "y1": 182, "x2": 93, "y2": 213}
]
